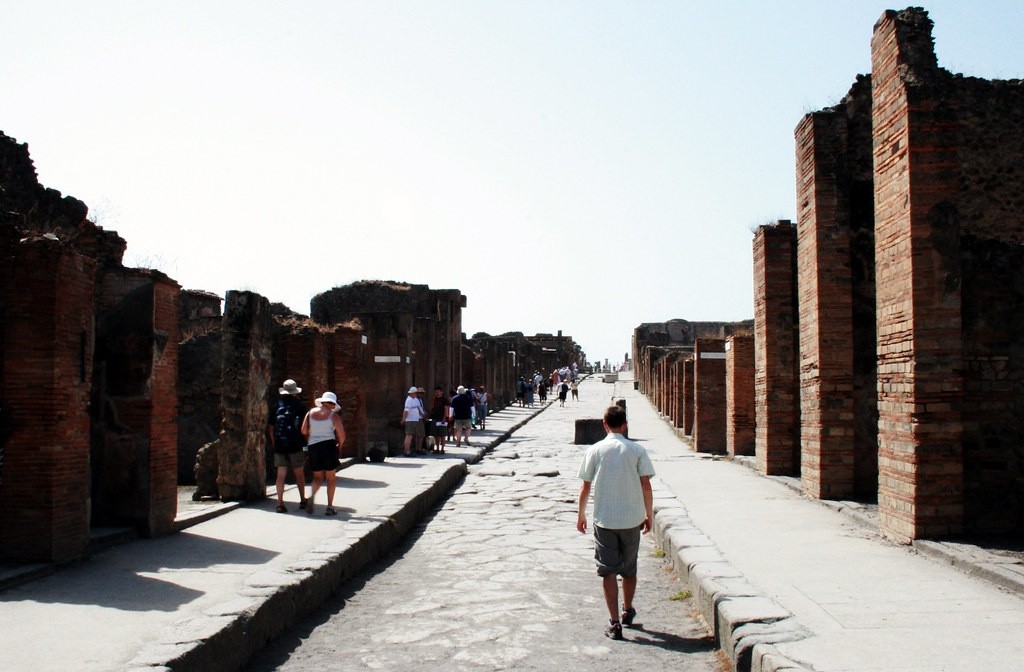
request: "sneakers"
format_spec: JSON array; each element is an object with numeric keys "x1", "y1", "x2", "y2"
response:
[
  {"x1": 621, "y1": 602, "x2": 636, "y2": 626},
  {"x1": 602, "y1": 619, "x2": 622, "y2": 640}
]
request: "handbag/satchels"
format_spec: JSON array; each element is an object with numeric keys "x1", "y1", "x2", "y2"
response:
[
  {"x1": 528, "y1": 386, "x2": 532, "y2": 391},
  {"x1": 521, "y1": 382, "x2": 527, "y2": 394},
  {"x1": 562, "y1": 384, "x2": 567, "y2": 392}
]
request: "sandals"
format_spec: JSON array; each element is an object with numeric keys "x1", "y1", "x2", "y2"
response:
[
  {"x1": 300, "y1": 499, "x2": 307, "y2": 509},
  {"x1": 276, "y1": 503, "x2": 288, "y2": 513}
]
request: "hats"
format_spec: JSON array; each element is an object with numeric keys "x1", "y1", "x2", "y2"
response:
[
  {"x1": 279, "y1": 379, "x2": 302, "y2": 394},
  {"x1": 418, "y1": 388, "x2": 425, "y2": 392},
  {"x1": 457, "y1": 386, "x2": 466, "y2": 394},
  {"x1": 408, "y1": 387, "x2": 417, "y2": 393},
  {"x1": 315, "y1": 392, "x2": 341, "y2": 412}
]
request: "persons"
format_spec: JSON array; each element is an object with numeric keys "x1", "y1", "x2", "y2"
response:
[
  {"x1": 576, "y1": 406, "x2": 654, "y2": 638},
  {"x1": 533, "y1": 362, "x2": 578, "y2": 407},
  {"x1": 401, "y1": 385, "x2": 492, "y2": 457},
  {"x1": 516, "y1": 377, "x2": 535, "y2": 408},
  {"x1": 595, "y1": 365, "x2": 597, "y2": 372},
  {"x1": 268, "y1": 380, "x2": 308, "y2": 513},
  {"x1": 302, "y1": 392, "x2": 345, "y2": 514}
]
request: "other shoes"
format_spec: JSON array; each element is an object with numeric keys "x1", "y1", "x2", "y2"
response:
[
  {"x1": 416, "y1": 450, "x2": 427, "y2": 455},
  {"x1": 307, "y1": 497, "x2": 314, "y2": 514},
  {"x1": 325, "y1": 507, "x2": 337, "y2": 515},
  {"x1": 404, "y1": 451, "x2": 417, "y2": 457}
]
